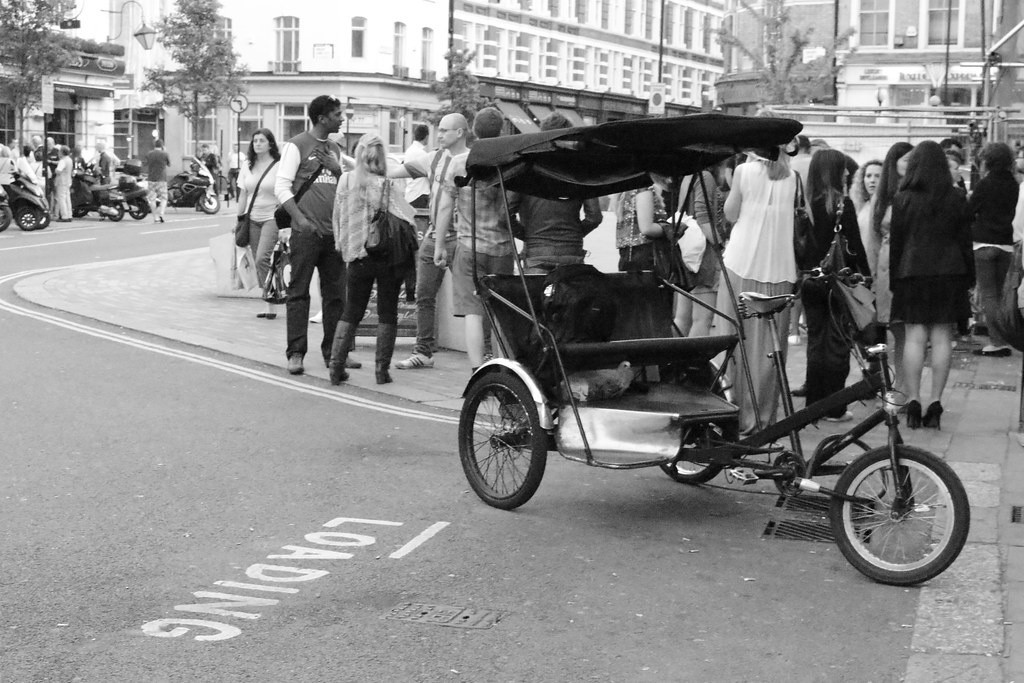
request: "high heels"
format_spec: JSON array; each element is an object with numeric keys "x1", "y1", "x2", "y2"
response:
[
  {"x1": 904, "y1": 400, "x2": 921, "y2": 429},
  {"x1": 922, "y1": 400, "x2": 943, "y2": 429}
]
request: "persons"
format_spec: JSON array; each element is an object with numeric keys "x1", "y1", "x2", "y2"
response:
[{"x1": 0, "y1": 92, "x2": 1024, "y2": 452}]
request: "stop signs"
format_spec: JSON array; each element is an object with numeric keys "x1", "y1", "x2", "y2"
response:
[{"x1": 345, "y1": 101, "x2": 354, "y2": 119}]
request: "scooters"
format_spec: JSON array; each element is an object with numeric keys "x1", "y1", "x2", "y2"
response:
[{"x1": 0, "y1": 155, "x2": 221, "y2": 231}]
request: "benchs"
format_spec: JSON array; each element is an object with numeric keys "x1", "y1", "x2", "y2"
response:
[{"x1": 488, "y1": 264, "x2": 742, "y2": 400}]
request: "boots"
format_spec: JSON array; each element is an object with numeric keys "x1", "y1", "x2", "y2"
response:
[
  {"x1": 374, "y1": 322, "x2": 397, "y2": 385},
  {"x1": 329, "y1": 320, "x2": 359, "y2": 385}
]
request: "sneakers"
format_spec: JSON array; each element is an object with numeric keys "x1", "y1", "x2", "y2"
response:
[
  {"x1": 395, "y1": 353, "x2": 435, "y2": 369},
  {"x1": 324, "y1": 354, "x2": 362, "y2": 369},
  {"x1": 309, "y1": 310, "x2": 322, "y2": 323},
  {"x1": 286, "y1": 354, "x2": 305, "y2": 373}
]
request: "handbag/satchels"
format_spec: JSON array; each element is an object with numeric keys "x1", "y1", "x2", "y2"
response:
[
  {"x1": 261, "y1": 240, "x2": 293, "y2": 305},
  {"x1": 274, "y1": 201, "x2": 294, "y2": 229},
  {"x1": 802, "y1": 195, "x2": 859, "y2": 312},
  {"x1": 791, "y1": 168, "x2": 820, "y2": 269},
  {"x1": 364, "y1": 176, "x2": 408, "y2": 283},
  {"x1": 234, "y1": 212, "x2": 251, "y2": 248},
  {"x1": 207, "y1": 232, "x2": 263, "y2": 298}
]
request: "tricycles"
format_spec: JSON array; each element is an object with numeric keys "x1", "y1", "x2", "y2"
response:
[{"x1": 459, "y1": 112, "x2": 972, "y2": 587}]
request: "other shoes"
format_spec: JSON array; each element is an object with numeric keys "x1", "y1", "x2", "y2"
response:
[
  {"x1": 828, "y1": 409, "x2": 854, "y2": 423},
  {"x1": 154, "y1": 219, "x2": 160, "y2": 223},
  {"x1": 1001, "y1": 347, "x2": 1013, "y2": 358},
  {"x1": 55, "y1": 217, "x2": 72, "y2": 222},
  {"x1": 256, "y1": 312, "x2": 266, "y2": 318},
  {"x1": 790, "y1": 384, "x2": 807, "y2": 397},
  {"x1": 971, "y1": 347, "x2": 1004, "y2": 357},
  {"x1": 266, "y1": 313, "x2": 277, "y2": 319},
  {"x1": 159, "y1": 215, "x2": 164, "y2": 223},
  {"x1": 738, "y1": 434, "x2": 784, "y2": 453}
]
